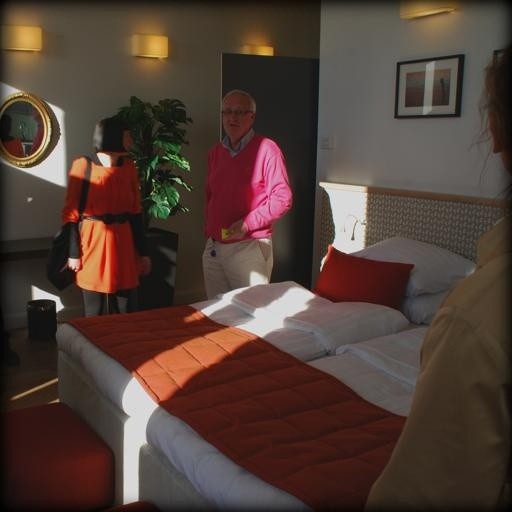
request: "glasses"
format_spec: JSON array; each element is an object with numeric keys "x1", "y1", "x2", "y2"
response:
[{"x1": 221, "y1": 107, "x2": 255, "y2": 118}]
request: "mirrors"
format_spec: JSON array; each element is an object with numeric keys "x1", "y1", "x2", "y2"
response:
[{"x1": 1, "y1": 91, "x2": 57, "y2": 170}]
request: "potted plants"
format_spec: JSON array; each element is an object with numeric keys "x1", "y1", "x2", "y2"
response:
[{"x1": 108, "y1": 93, "x2": 202, "y2": 313}]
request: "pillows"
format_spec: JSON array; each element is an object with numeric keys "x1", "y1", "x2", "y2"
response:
[
  {"x1": 401, "y1": 288, "x2": 457, "y2": 329},
  {"x1": 344, "y1": 233, "x2": 480, "y2": 299},
  {"x1": 312, "y1": 242, "x2": 417, "y2": 316}
]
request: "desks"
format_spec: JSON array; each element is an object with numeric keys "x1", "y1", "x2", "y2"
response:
[{"x1": 0, "y1": 227, "x2": 178, "y2": 310}]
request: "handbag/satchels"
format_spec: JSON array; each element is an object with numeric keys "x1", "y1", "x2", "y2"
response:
[{"x1": 48, "y1": 224, "x2": 76, "y2": 276}]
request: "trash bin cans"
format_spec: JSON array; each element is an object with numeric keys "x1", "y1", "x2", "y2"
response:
[{"x1": 26, "y1": 300, "x2": 56, "y2": 341}]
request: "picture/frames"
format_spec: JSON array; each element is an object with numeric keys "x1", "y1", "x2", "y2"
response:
[
  {"x1": 392, "y1": 51, "x2": 464, "y2": 119},
  {"x1": 494, "y1": 48, "x2": 504, "y2": 63}
]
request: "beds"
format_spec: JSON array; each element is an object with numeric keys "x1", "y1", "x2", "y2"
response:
[
  {"x1": 131, "y1": 322, "x2": 424, "y2": 511},
  {"x1": 49, "y1": 279, "x2": 412, "y2": 505}
]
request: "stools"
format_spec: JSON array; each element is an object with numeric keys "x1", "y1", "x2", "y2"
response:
[
  {"x1": 1, "y1": 397, "x2": 118, "y2": 511},
  {"x1": 103, "y1": 498, "x2": 158, "y2": 512}
]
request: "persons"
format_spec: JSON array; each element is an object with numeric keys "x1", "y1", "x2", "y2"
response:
[
  {"x1": 364, "y1": 43, "x2": 512, "y2": 512},
  {"x1": 60, "y1": 118, "x2": 152, "y2": 317},
  {"x1": 202, "y1": 91, "x2": 293, "y2": 300}
]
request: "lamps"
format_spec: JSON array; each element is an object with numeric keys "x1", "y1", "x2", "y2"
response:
[
  {"x1": 242, "y1": 45, "x2": 274, "y2": 56},
  {"x1": 131, "y1": 35, "x2": 169, "y2": 59},
  {"x1": 1, "y1": 24, "x2": 44, "y2": 53}
]
